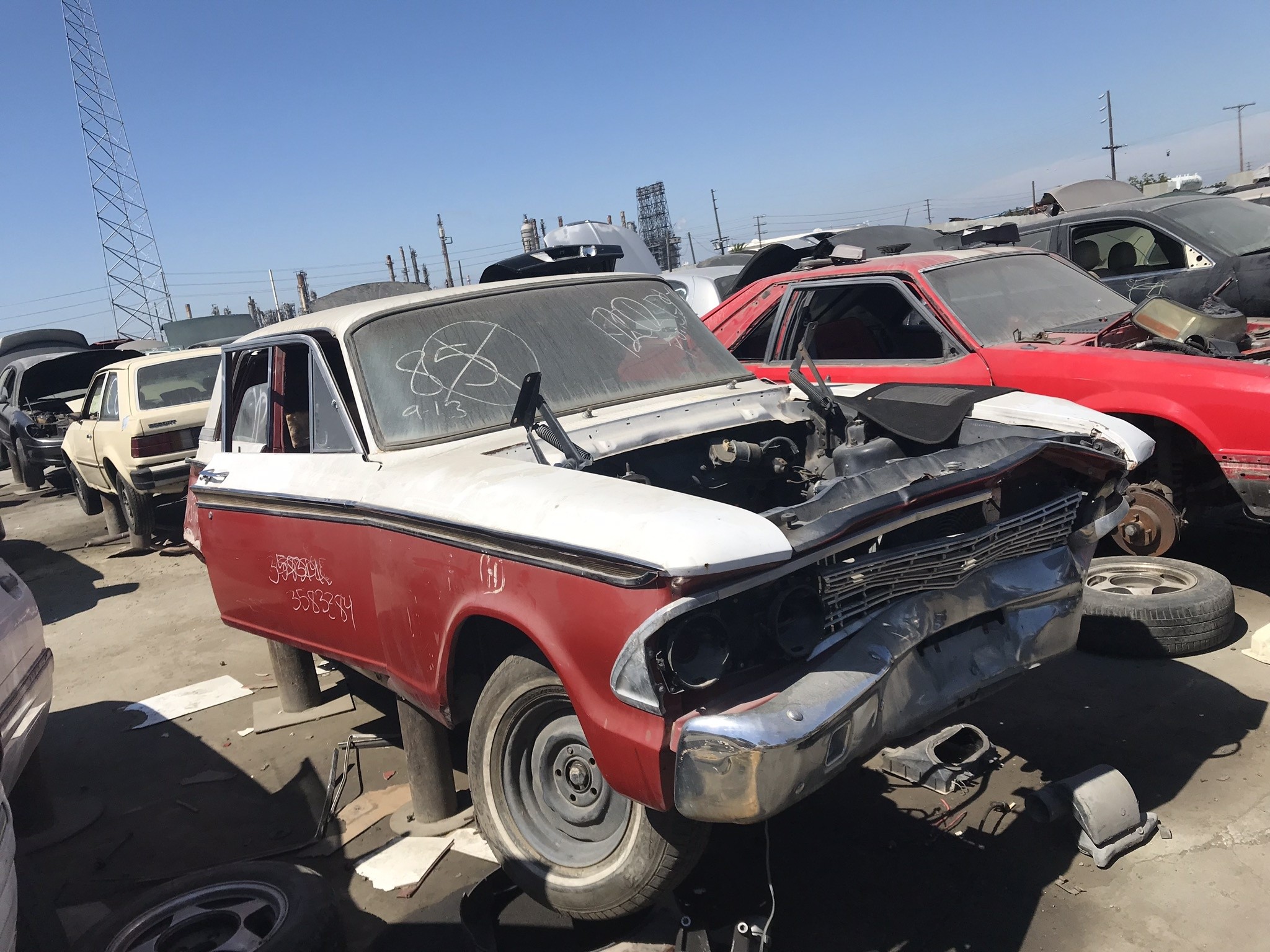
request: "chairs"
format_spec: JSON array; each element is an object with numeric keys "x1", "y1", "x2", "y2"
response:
[
  {"x1": 1074, "y1": 239, "x2": 1153, "y2": 278},
  {"x1": 801, "y1": 316, "x2": 886, "y2": 358},
  {"x1": 160, "y1": 387, "x2": 201, "y2": 405}
]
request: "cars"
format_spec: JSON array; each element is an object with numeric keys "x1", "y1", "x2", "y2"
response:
[
  {"x1": 0, "y1": 558, "x2": 57, "y2": 800},
  {"x1": 955, "y1": 188, "x2": 1270, "y2": 322},
  {"x1": 0, "y1": 231, "x2": 754, "y2": 552},
  {"x1": 619, "y1": 242, "x2": 1270, "y2": 573},
  {"x1": 176, "y1": 269, "x2": 1157, "y2": 927}
]
